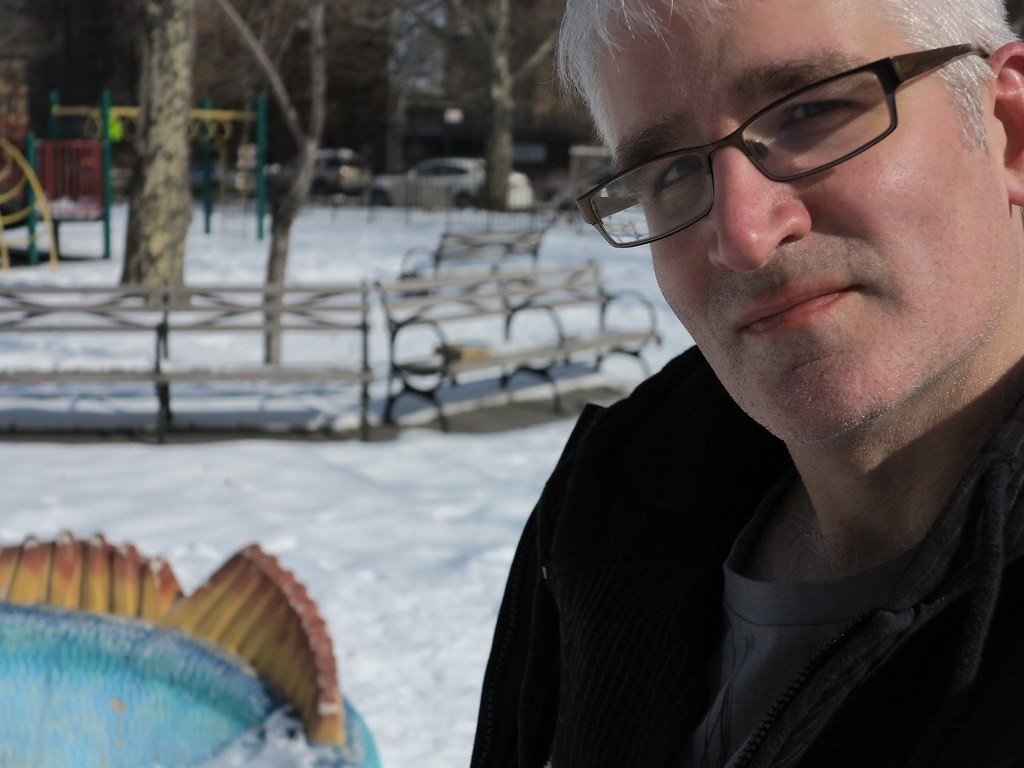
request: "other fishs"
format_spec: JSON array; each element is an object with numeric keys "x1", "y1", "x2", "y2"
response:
[{"x1": 0, "y1": 531, "x2": 381, "y2": 768}]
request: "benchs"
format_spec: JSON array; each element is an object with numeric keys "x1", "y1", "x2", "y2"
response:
[
  {"x1": 0, "y1": 279, "x2": 393, "y2": 444},
  {"x1": 402, "y1": 213, "x2": 565, "y2": 283},
  {"x1": 371, "y1": 255, "x2": 668, "y2": 433}
]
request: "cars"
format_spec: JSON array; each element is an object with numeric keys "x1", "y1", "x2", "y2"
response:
[
  {"x1": 371, "y1": 155, "x2": 535, "y2": 213},
  {"x1": 289, "y1": 146, "x2": 378, "y2": 201}
]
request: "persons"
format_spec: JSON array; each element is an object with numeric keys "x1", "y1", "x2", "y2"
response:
[{"x1": 471, "y1": 0, "x2": 1024, "y2": 767}]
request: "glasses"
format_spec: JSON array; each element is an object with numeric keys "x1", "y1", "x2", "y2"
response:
[{"x1": 576, "y1": 42, "x2": 988, "y2": 250}]
